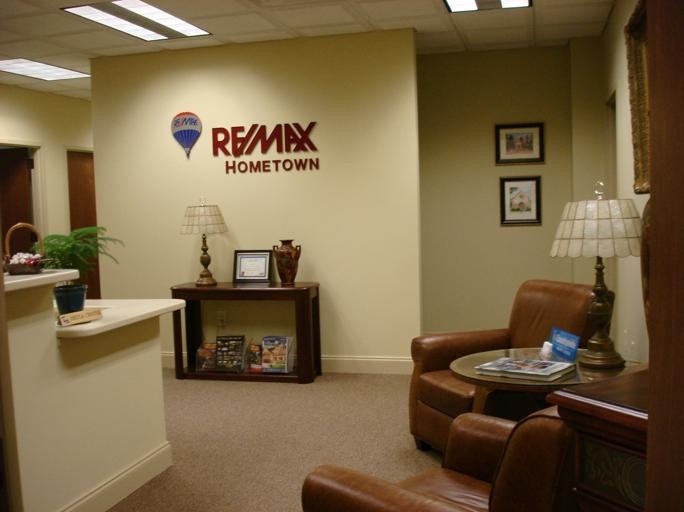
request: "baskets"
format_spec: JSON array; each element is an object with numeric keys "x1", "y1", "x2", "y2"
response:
[{"x1": 7, "y1": 223, "x2": 45, "y2": 274}]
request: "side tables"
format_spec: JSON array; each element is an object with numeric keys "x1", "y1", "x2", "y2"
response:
[{"x1": 449, "y1": 348, "x2": 643, "y2": 414}]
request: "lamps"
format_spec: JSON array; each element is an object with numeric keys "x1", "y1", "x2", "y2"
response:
[
  {"x1": 549, "y1": 198, "x2": 643, "y2": 370},
  {"x1": 180, "y1": 205, "x2": 228, "y2": 286}
]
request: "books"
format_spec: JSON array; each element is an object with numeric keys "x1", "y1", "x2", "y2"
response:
[
  {"x1": 474, "y1": 358, "x2": 576, "y2": 381},
  {"x1": 197, "y1": 335, "x2": 294, "y2": 374}
]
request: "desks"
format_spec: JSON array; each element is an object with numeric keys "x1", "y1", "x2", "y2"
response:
[
  {"x1": 170, "y1": 282, "x2": 321, "y2": 384},
  {"x1": 544, "y1": 369, "x2": 650, "y2": 512}
]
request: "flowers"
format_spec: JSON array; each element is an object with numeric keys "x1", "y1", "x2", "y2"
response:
[{"x1": 9, "y1": 252, "x2": 41, "y2": 265}]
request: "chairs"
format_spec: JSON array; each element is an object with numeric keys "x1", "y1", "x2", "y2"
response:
[
  {"x1": 300, "y1": 405, "x2": 569, "y2": 512},
  {"x1": 408, "y1": 278, "x2": 615, "y2": 454}
]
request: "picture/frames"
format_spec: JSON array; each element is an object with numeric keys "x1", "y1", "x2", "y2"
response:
[
  {"x1": 499, "y1": 174, "x2": 542, "y2": 225},
  {"x1": 233, "y1": 250, "x2": 272, "y2": 286},
  {"x1": 494, "y1": 122, "x2": 544, "y2": 164},
  {"x1": 623, "y1": 0, "x2": 653, "y2": 193}
]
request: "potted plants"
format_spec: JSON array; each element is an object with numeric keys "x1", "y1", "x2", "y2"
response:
[{"x1": 30, "y1": 226, "x2": 126, "y2": 315}]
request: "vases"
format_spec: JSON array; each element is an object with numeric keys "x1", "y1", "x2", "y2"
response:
[
  {"x1": 273, "y1": 239, "x2": 301, "y2": 285},
  {"x1": 7, "y1": 264, "x2": 41, "y2": 275}
]
